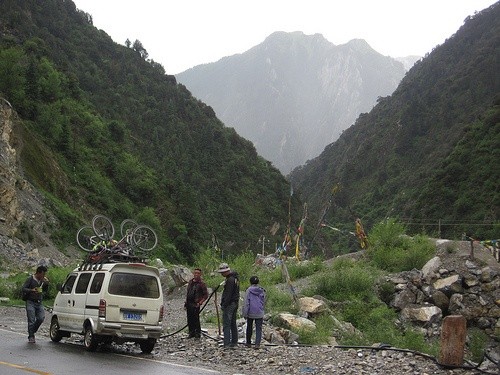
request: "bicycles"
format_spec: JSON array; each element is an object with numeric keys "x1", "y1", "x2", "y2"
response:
[{"x1": 73, "y1": 213, "x2": 158, "y2": 263}]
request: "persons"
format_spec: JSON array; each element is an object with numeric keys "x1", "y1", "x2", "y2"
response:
[
  {"x1": 184, "y1": 269, "x2": 208, "y2": 338},
  {"x1": 21, "y1": 266, "x2": 48, "y2": 342},
  {"x1": 242, "y1": 276, "x2": 267, "y2": 349},
  {"x1": 217, "y1": 263, "x2": 240, "y2": 348}
]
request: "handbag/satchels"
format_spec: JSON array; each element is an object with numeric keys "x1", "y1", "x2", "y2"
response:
[{"x1": 21, "y1": 292, "x2": 29, "y2": 301}]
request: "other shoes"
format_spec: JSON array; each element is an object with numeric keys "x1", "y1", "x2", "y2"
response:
[
  {"x1": 245, "y1": 344, "x2": 251, "y2": 347},
  {"x1": 28, "y1": 336, "x2": 36, "y2": 343},
  {"x1": 255, "y1": 346, "x2": 259, "y2": 350},
  {"x1": 194, "y1": 336, "x2": 200, "y2": 341},
  {"x1": 187, "y1": 334, "x2": 195, "y2": 339}
]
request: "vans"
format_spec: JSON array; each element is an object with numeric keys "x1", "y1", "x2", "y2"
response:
[{"x1": 49, "y1": 262, "x2": 165, "y2": 351}]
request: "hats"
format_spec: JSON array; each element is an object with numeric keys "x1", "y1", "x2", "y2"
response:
[
  {"x1": 250, "y1": 276, "x2": 259, "y2": 284},
  {"x1": 217, "y1": 263, "x2": 230, "y2": 273}
]
